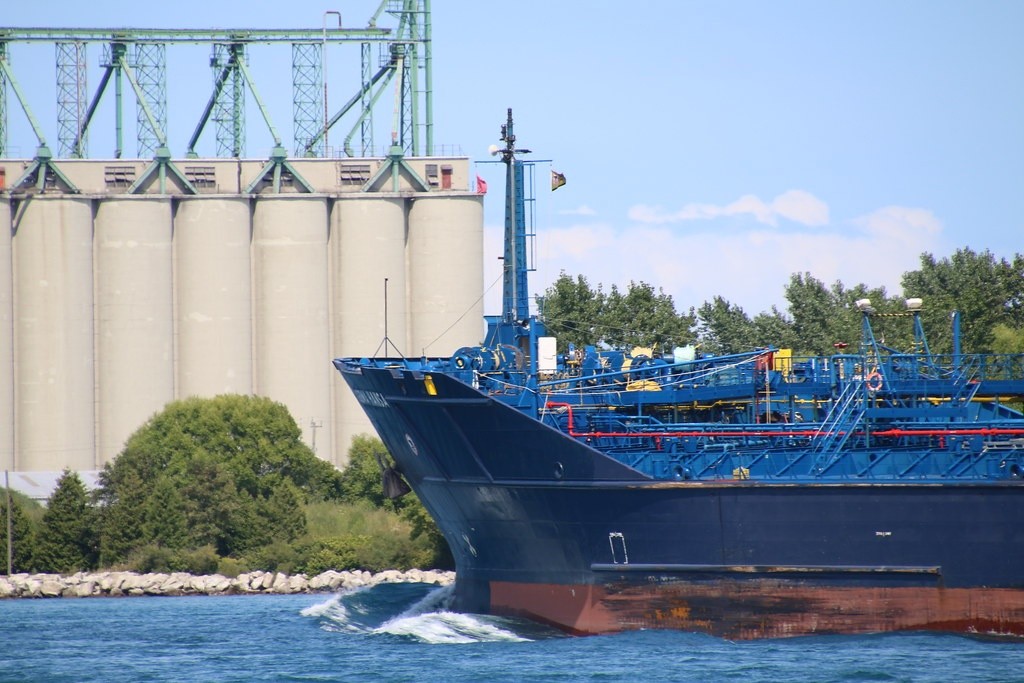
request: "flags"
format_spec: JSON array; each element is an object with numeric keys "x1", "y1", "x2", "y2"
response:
[
  {"x1": 552, "y1": 170, "x2": 566, "y2": 192},
  {"x1": 477, "y1": 175, "x2": 487, "y2": 194}
]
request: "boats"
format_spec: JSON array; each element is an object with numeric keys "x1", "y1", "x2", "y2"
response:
[{"x1": 330, "y1": 106, "x2": 1024, "y2": 639}]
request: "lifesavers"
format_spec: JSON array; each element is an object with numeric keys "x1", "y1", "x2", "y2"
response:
[{"x1": 865, "y1": 371, "x2": 884, "y2": 392}]
